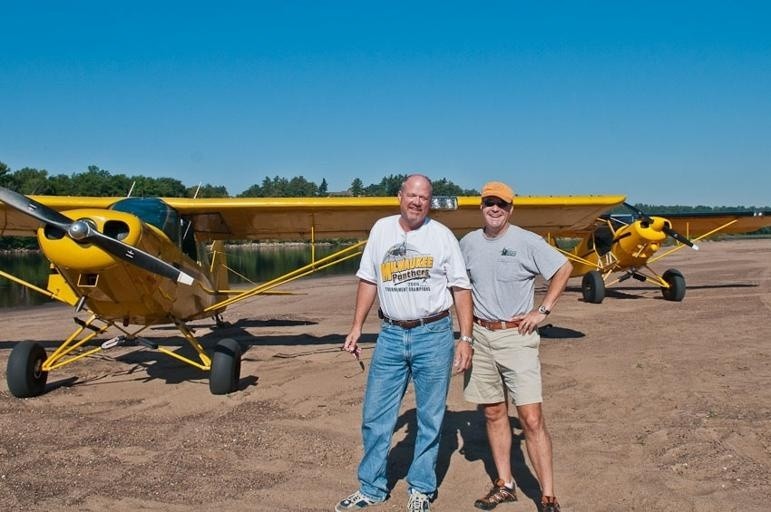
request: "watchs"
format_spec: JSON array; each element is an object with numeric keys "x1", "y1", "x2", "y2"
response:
[
  {"x1": 538, "y1": 305, "x2": 550, "y2": 316},
  {"x1": 459, "y1": 335, "x2": 472, "y2": 345}
]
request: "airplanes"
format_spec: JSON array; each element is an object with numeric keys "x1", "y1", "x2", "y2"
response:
[
  {"x1": 0, "y1": 179, "x2": 627, "y2": 397},
  {"x1": 546, "y1": 202, "x2": 771, "y2": 304}
]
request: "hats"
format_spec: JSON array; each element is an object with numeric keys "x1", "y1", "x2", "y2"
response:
[{"x1": 481, "y1": 182, "x2": 512, "y2": 204}]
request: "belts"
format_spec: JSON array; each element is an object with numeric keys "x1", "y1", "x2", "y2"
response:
[
  {"x1": 473, "y1": 316, "x2": 523, "y2": 330},
  {"x1": 383, "y1": 310, "x2": 449, "y2": 329}
]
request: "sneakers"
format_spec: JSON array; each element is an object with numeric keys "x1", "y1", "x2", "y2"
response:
[
  {"x1": 475, "y1": 478, "x2": 517, "y2": 510},
  {"x1": 335, "y1": 490, "x2": 383, "y2": 512},
  {"x1": 540, "y1": 494, "x2": 560, "y2": 512},
  {"x1": 407, "y1": 488, "x2": 430, "y2": 512}
]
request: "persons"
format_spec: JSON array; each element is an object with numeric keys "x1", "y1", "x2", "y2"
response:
[
  {"x1": 333, "y1": 175, "x2": 473, "y2": 512},
  {"x1": 456, "y1": 184, "x2": 572, "y2": 511}
]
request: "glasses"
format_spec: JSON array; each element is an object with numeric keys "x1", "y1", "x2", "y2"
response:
[
  {"x1": 485, "y1": 198, "x2": 507, "y2": 208},
  {"x1": 336, "y1": 344, "x2": 365, "y2": 378}
]
